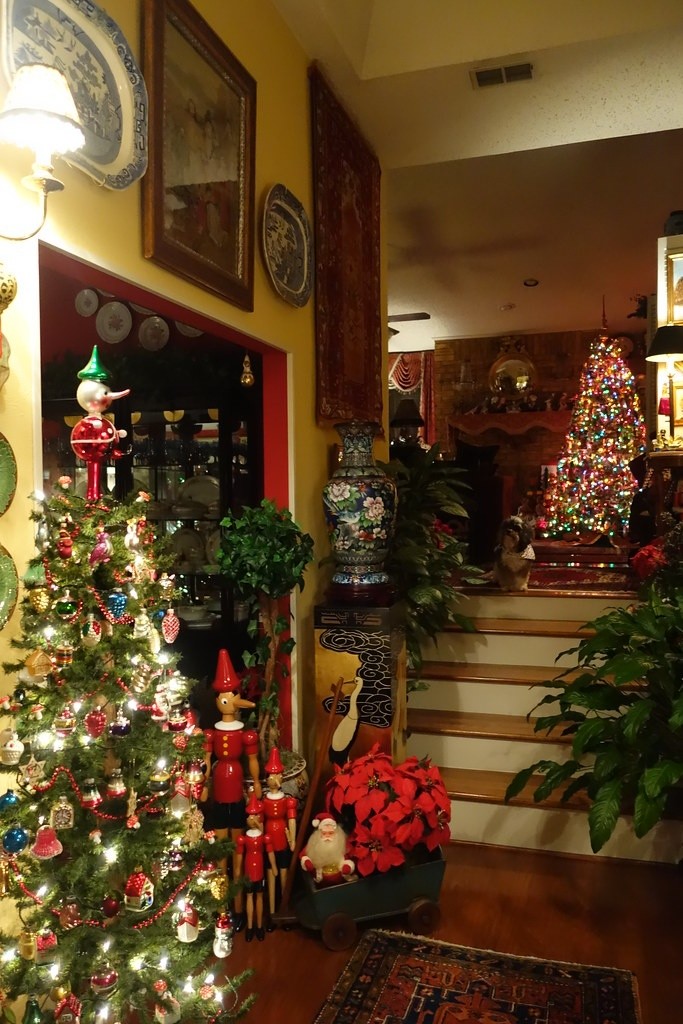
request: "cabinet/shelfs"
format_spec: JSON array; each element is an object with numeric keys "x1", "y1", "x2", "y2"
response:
[{"x1": 43, "y1": 379, "x2": 259, "y2": 641}]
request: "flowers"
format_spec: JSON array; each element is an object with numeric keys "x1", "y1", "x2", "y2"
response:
[
  {"x1": 376, "y1": 439, "x2": 490, "y2": 672},
  {"x1": 506, "y1": 545, "x2": 683, "y2": 854},
  {"x1": 322, "y1": 742, "x2": 452, "y2": 878}
]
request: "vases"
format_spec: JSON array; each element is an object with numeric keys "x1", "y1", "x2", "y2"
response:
[{"x1": 320, "y1": 420, "x2": 397, "y2": 609}]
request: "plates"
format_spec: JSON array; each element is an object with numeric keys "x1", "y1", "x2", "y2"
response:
[
  {"x1": 76, "y1": 289, "x2": 98, "y2": 317},
  {"x1": 129, "y1": 302, "x2": 157, "y2": 314},
  {"x1": 176, "y1": 321, "x2": 204, "y2": 337},
  {"x1": 96, "y1": 302, "x2": 132, "y2": 343},
  {"x1": 97, "y1": 288, "x2": 115, "y2": 297},
  {"x1": 1, "y1": 0, "x2": 149, "y2": 190},
  {"x1": 262, "y1": 183, "x2": 314, "y2": 307},
  {"x1": 206, "y1": 530, "x2": 222, "y2": 565},
  {"x1": 170, "y1": 528, "x2": 203, "y2": 561},
  {"x1": 138, "y1": 316, "x2": 169, "y2": 352},
  {"x1": 176, "y1": 475, "x2": 219, "y2": 503}
]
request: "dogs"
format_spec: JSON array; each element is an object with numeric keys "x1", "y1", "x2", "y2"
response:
[{"x1": 476, "y1": 515, "x2": 535, "y2": 591}]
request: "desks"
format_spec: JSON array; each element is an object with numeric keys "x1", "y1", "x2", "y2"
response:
[
  {"x1": 643, "y1": 455, "x2": 683, "y2": 537},
  {"x1": 445, "y1": 410, "x2": 572, "y2": 461}
]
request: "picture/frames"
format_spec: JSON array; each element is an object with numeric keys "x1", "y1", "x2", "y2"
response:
[
  {"x1": 141, "y1": 0, "x2": 258, "y2": 313},
  {"x1": 306, "y1": 62, "x2": 385, "y2": 440},
  {"x1": 666, "y1": 252, "x2": 683, "y2": 326},
  {"x1": 673, "y1": 382, "x2": 683, "y2": 427}
]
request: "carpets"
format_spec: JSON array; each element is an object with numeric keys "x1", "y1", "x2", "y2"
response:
[{"x1": 311, "y1": 928, "x2": 643, "y2": 1024}]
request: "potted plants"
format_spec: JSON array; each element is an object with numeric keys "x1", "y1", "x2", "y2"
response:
[{"x1": 197, "y1": 498, "x2": 314, "y2": 833}]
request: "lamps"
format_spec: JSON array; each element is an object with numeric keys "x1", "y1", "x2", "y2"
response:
[
  {"x1": 644, "y1": 326, "x2": 683, "y2": 448},
  {"x1": 0, "y1": 61, "x2": 87, "y2": 242},
  {"x1": 390, "y1": 398, "x2": 424, "y2": 430}
]
